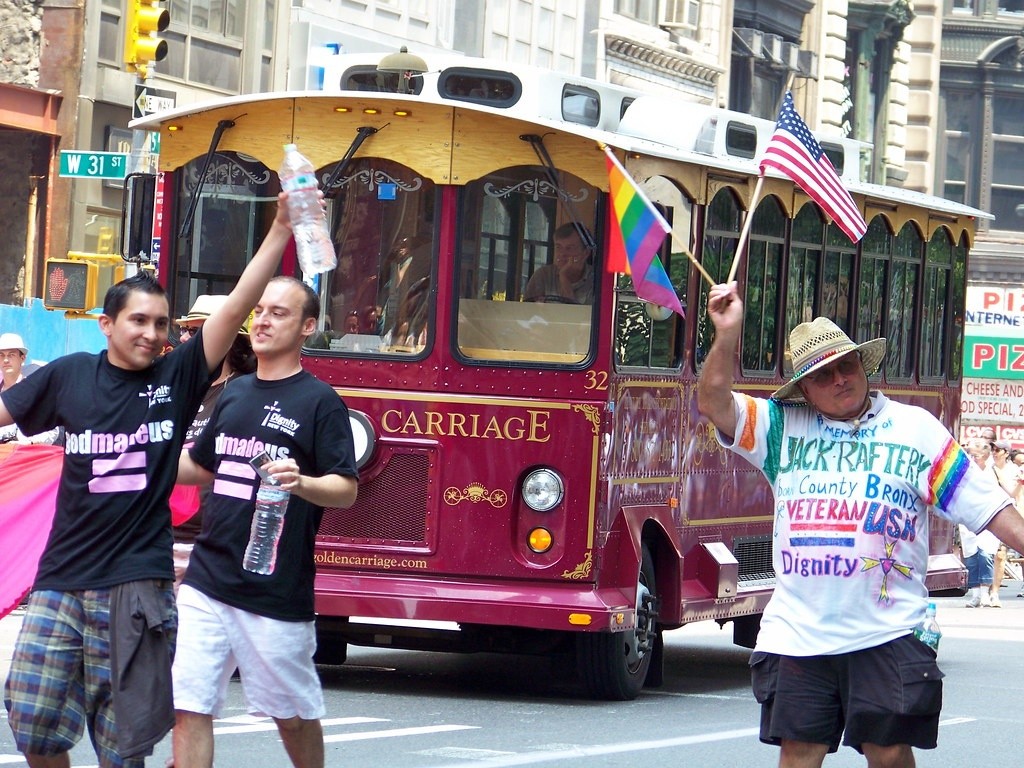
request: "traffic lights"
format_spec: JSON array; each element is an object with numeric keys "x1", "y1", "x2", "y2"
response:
[{"x1": 122, "y1": 1, "x2": 170, "y2": 67}]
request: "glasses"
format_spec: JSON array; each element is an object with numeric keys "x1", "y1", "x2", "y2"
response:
[
  {"x1": 805, "y1": 355, "x2": 859, "y2": 388},
  {"x1": 992, "y1": 448, "x2": 1001, "y2": 453},
  {"x1": 179, "y1": 326, "x2": 199, "y2": 337}
]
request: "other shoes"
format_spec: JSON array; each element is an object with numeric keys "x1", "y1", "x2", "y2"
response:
[
  {"x1": 966, "y1": 586, "x2": 1001, "y2": 608},
  {"x1": 1017, "y1": 589, "x2": 1024, "y2": 597}
]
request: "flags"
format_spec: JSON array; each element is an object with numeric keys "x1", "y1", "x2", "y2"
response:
[
  {"x1": 758, "y1": 89, "x2": 868, "y2": 244},
  {"x1": 605, "y1": 151, "x2": 685, "y2": 318}
]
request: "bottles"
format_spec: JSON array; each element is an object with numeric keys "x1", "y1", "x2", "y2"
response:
[
  {"x1": 913, "y1": 603, "x2": 939, "y2": 655},
  {"x1": 278, "y1": 144, "x2": 337, "y2": 276},
  {"x1": 242, "y1": 479, "x2": 290, "y2": 575}
]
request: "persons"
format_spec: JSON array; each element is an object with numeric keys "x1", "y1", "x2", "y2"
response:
[
  {"x1": 365, "y1": 306, "x2": 382, "y2": 332},
  {"x1": 959, "y1": 430, "x2": 1024, "y2": 608},
  {"x1": 169, "y1": 295, "x2": 258, "y2": 597},
  {"x1": 376, "y1": 237, "x2": 431, "y2": 348},
  {"x1": 0, "y1": 178, "x2": 328, "y2": 768},
  {"x1": 344, "y1": 311, "x2": 359, "y2": 334},
  {"x1": 697, "y1": 281, "x2": 1024, "y2": 768},
  {"x1": 172, "y1": 277, "x2": 360, "y2": 768},
  {"x1": 0, "y1": 333, "x2": 60, "y2": 447},
  {"x1": 522, "y1": 222, "x2": 595, "y2": 305}
]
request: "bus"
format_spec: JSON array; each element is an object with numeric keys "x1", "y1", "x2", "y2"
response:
[{"x1": 124, "y1": 49, "x2": 997, "y2": 702}]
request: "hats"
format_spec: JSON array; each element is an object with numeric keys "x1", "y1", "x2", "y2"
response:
[
  {"x1": 772, "y1": 317, "x2": 886, "y2": 401},
  {"x1": 0, "y1": 333, "x2": 29, "y2": 355},
  {"x1": 989, "y1": 441, "x2": 1009, "y2": 450},
  {"x1": 176, "y1": 295, "x2": 249, "y2": 334}
]
